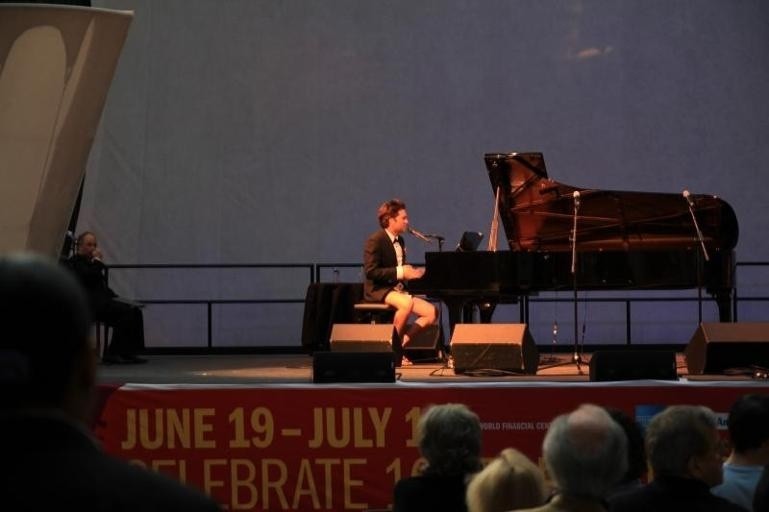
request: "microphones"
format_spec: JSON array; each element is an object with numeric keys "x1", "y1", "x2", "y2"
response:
[
  {"x1": 574, "y1": 191, "x2": 581, "y2": 208},
  {"x1": 407, "y1": 226, "x2": 434, "y2": 246},
  {"x1": 683, "y1": 190, "x2": 694, "y2": 207}
]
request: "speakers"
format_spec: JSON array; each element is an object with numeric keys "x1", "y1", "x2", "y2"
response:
[
  {"x1": 450, "y1": 323, "x2": 539, "y2": 374},
  {"x1": 330, "y1": 324, "x2": 404, "y2": 362},
  {"x1": 686, "y1": 322, "x2": 769, "y2": 373},
  {"x1": 591, "y1": 347, "x2": 679, "y2": 381},
  {"x1": 405, "y1": 321, "x2": 446, "y2": 362}
]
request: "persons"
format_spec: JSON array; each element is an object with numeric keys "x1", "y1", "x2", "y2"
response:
[
  {"x1": 393, "y1": 390, "x2": 767, "y2": 511},
  {"x1": 68, "y1": 232, "x2": 146, "y2": 365},
  {"x1": 362, "y1": 199, "x2": 439, "y2": 366},
  {"x1": 2, "y1": 254, "x2": 224, "y2": 511}
]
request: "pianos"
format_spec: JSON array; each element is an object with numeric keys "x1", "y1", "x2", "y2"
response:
[{"x1": 424, "y1": 151, "x2": 740, "y2": 323}]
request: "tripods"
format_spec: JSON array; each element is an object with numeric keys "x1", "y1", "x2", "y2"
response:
[{"x1": 537, "y1": 270, "x2": 589, "y2": 375}]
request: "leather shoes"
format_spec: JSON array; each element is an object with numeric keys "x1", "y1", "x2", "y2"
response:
[{"x1": 101, "y1": 352, "x2": 149, "y2": 365}]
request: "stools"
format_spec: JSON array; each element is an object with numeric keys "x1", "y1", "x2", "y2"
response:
[
  {"x1": 355, "y1": 303, "x2": 397, "y2": 325},
  {"x1": 86, "y1": 318, "x2": 112, "y2": 360}
]
